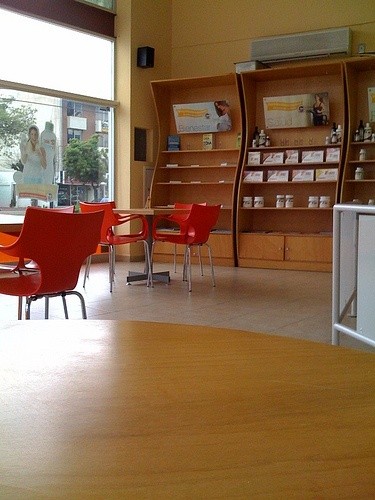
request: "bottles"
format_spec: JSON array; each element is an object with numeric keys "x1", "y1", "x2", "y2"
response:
[
  {"x1": 360, "y1": 149, "x2": 366, "y2": 161},
  {"x1": 251, "y1": 126, "x2": 271, "y2": 147},
  {"x1": 243, "y1": 196, "x2": 264, "y2": 209},
  {"x1": 354, "y1": 168, "x2": 365, "y2": 180},
  {"x1": 354, "y1": 120, "x2": 375, "y2": 142},
  {"x1": 325, "y1": 122, "x2": 342, "y2": 145},
  {"x1": 307, "y1": 195, "x2": 331, "y2": 208},
  {"x1": 276, "y1": 194, "x2": 294, "y2": 208}
]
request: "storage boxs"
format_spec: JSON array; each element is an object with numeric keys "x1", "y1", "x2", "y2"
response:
[
  {"x1": 168, "y1": 135, "x2": 180, "y2": 151},
  {"x1": 236, "y1": 132, "x2": 241, "y2": 146},
  {"x1": 203, "y1": 134, "x2": 215, "y2": 149},
  {"x1": 233, "y1": 60, "x2": 270, "y2": 74}
]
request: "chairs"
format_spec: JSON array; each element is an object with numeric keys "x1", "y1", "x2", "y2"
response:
[{"x1": 0, "y1": 201, "x2": 222, "y2": 319}]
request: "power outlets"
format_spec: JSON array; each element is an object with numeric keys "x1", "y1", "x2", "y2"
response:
[{"x1": 358, "y1": 44, "x2": 364, "y2": 53}]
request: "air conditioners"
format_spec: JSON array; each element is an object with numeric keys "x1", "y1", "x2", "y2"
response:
[{"x1": 251, "y1": 28, "x2": 353, "y2": 65}]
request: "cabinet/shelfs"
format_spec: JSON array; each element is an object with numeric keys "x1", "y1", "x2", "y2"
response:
[
  {"x1": 148, "y1": 72, "x2": 242, "y2": 265},
  {"x1": 340, "y1": 56, "x2": 375, "y2": 205},
  {"x1": 235, "y1": 61, "x2": 348, "y2": 271}
]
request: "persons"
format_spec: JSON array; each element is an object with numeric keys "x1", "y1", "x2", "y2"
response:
[
  {"x1": 313, "y1": 95, "x2": 325, "y2": 126},
  {"x1": 20, "y1": 127, "x2": 48, "y2": 184},
  {"x1": 214, "y1": 101, "x2": 232, "y2": 131}
]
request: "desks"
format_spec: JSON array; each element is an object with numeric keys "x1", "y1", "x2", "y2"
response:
[
  {"x1": 113, "y1": 208, "x2": 190, "y2": 283},
  {"x1": 0, "y1": 319, "x2": 375, "y2": 500},
  {"x1": 0, "y1": 214, "x2": 25, "y2": 236}
]
